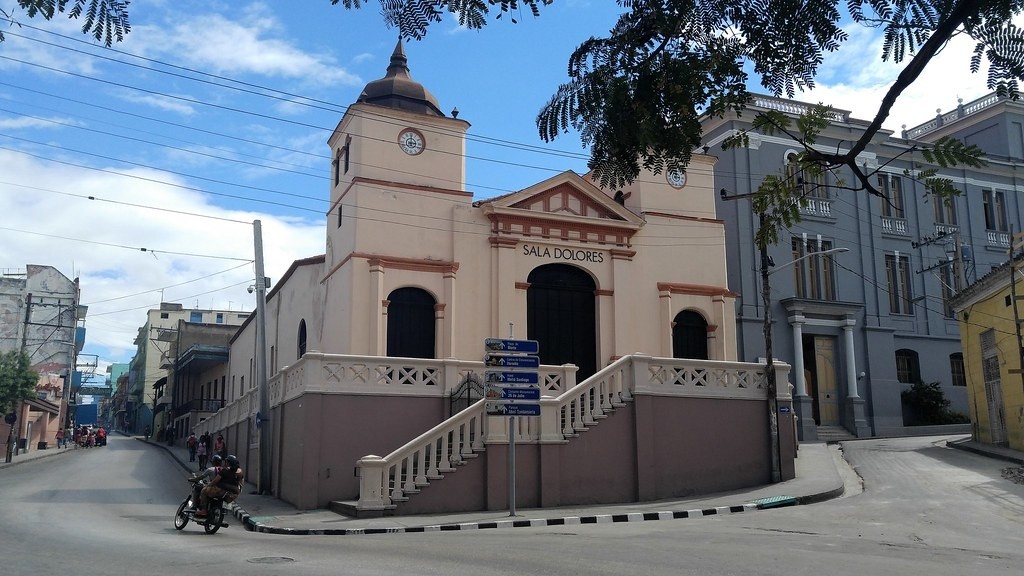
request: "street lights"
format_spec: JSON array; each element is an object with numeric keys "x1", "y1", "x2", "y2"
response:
[{"x1": 758, "y1": 248, "x2": 853, "y2": 484}]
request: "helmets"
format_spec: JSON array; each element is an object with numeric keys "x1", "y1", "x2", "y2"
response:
[
  {"x1": 210, "y1": 455, "x2": 222, "y2": 463},
  {"x1": 225, "y1": 455, "x2": 237, "y2": 462}
]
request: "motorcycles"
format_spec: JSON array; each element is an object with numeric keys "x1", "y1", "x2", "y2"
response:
[{"x1": 174, "y1": 472, "x2": 230, "y2": 536}]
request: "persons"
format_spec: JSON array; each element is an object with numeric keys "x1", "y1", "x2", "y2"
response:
[
  {"x1": 195, "y1": 455, "x2": 244, "y2": 515},
  {"x1": 144, "y1": 422, "x2": 175, "y2": 439},
  {"x1": 55, "y1": 425, "x2": 107, "y2": 450},
  {"x1": 186, "y1": 431, "x2": 225, "y2": 470}
]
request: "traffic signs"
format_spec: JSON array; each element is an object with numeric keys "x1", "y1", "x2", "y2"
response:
[
  {"x1": 485, "y1": 338, "x2": 539, "y2": 354},
  {"x1": 484, "y1": 354, "x2": 540, "y2": 370},
  {"x1": 485, "y1": 401, "x2": 542, "y2": 417},
  {"x1": 485, "y1": 385, "x2": 541, "y2": 400},
  {"x1": 483, "y1": 371, "x2": 538, "y2": 384}
]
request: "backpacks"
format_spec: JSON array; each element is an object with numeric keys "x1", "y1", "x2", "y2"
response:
[{"x1": 189, "y1": 435, "x2": 195, "y2": 446}]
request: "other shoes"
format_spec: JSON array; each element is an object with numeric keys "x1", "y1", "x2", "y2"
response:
[
  {"x1": 202, "y1": 467, "x2": 204, "y2": 471},
  {"x1": 190, "y1": 507, "x2": 197, "y2": 510},
  {"x1": 204, "y1": 466, "x2": 207, "y2": 469},
  {"x1": 199, "y1": 467, "x2": 201, "y2": 471},
  {"x1": 195, "y1": 509, "x2": 207, "y2": 516}
]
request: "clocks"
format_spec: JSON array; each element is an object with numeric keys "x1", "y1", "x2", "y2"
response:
[
  {"x1": 666, "y1": 167, "x2": 687, "y2": 189},
  {"x1": 397, "y1": 127, "x2": 426, "y2": 156}
]
